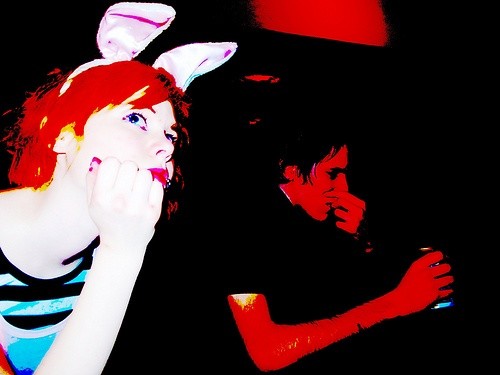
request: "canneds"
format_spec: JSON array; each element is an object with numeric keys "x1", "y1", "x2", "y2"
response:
[{"x1": 416, "y1": 247, "x2": 456, "y2": 309}]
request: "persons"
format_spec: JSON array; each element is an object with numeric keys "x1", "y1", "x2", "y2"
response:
[
  {"x1": 0, "y1": 2, "x2": 239, "y2": 375},
  {"x1": 227, "y1": 116, "x2": 454, "y2": 375}
]
